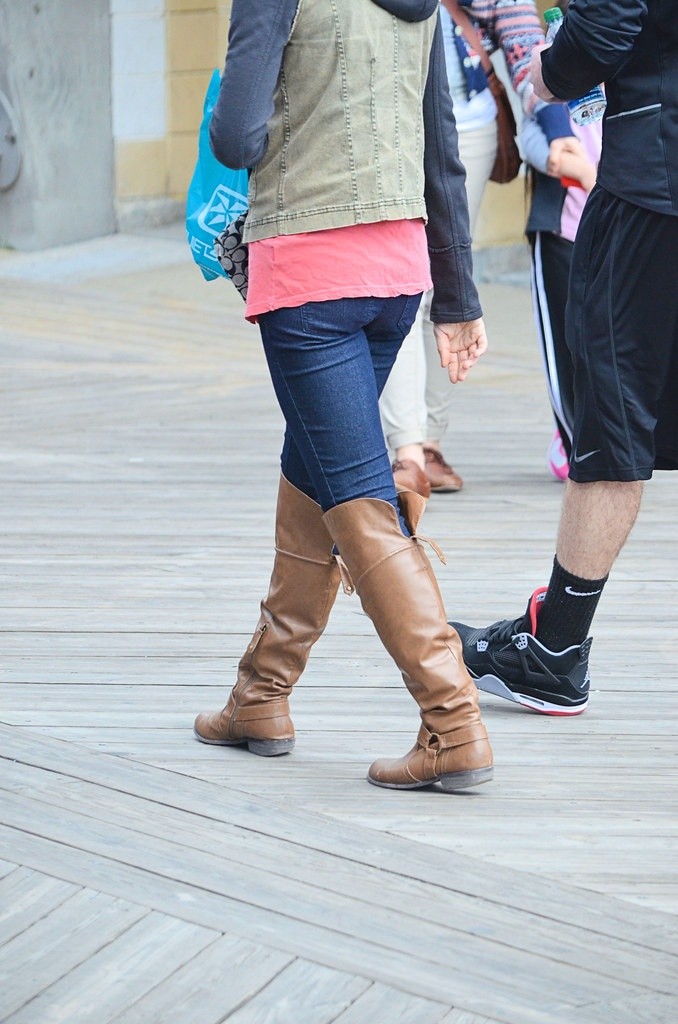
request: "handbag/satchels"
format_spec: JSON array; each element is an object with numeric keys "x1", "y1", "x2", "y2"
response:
[
  {"x1": 184, "y1": 64, "x2": 253, "y2": 285},
  {"x1": 481, "y1": 71, "x2": 524, "y2": 185},
  {"x1": 211, "y1": 209, "x2": 257, "y2": 305}
]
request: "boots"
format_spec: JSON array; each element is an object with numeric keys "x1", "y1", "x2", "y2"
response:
[
  {"x1": 321, "y1": 485, "x2": 496, "y2": 794},
  {"x1": 193, "y1": 465, "x2": 355, "y2": 758}
]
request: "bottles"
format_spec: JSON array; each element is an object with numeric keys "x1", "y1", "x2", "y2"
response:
[{"x1": 542, "y1": 6, "x2": 607, "y2": 126}]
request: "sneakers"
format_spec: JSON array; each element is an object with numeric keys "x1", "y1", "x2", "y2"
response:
[
  {"x1": 546, "y1": 422, "x2": 570, "y2": 480},
  {"x1": 444, "y1": 618, "x2": 597, "y2": 721}
]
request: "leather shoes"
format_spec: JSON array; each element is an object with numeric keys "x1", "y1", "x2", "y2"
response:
[
  {"x1": 423, "y1": 446, "x2": 465, "y2": 496},
  {"x1": 388, "y1": 458, "x2": 433, "y2": 502}
]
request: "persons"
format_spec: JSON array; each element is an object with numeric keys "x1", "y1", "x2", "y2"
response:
[
  {"x1": 193, "y1": 0, "x2": 497, "y2": 795},
  {"x1": 442, "y1": 0, "x2": 677, "y2": 720},
  {"x1": 373, "y1": 2, "x2": 608, "y2": 494}
]
